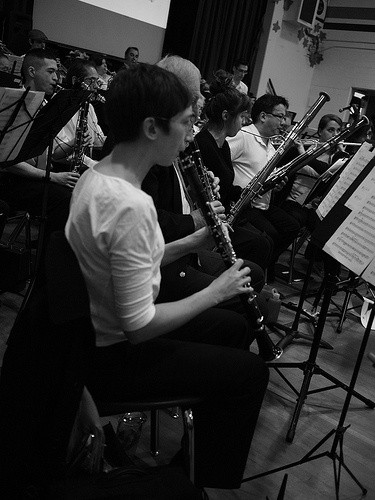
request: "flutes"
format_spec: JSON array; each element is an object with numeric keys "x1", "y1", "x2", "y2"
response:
[
  {"x1": 180, "y1": 151, "x2": 283, "y2": 362},
  {"x1": 191, "y1": 140, "x2": 236, "y2": 259}
]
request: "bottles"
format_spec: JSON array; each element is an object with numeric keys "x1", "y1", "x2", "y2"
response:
[
  {"x1": 117, "y1": 410, "x2": 147, "y2": 458},
  {"x1": 268, "y1": 288, "x2": 281, "y2": 323}
]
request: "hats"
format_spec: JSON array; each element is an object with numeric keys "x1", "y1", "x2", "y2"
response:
[{"x1": 27, "y1": 29, "x2": 48, "y2": 42}]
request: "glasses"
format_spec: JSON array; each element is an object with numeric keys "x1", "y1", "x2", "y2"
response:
[
  {"x1": 156, "y1": 114, "x2": 195, "y2": 130},
  {"x1": 236, "y1": 66, "x2": 248, "y2": 75},
  {"x1": 267, "y1": 112, "x2": 289, "y2": 122}
]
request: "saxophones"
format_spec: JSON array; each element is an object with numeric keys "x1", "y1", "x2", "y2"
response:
[{"x1": 52, "y1": 83, "x2": 87, "y2": 172}]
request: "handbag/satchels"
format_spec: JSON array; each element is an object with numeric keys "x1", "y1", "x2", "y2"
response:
[{"x1": 43, "y1": 467, "x2": 172, "y2": 500}]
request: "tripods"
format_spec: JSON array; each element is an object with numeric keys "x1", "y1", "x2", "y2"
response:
[
  {"x1": 267, "y1": 262, "x2": 375, "y2": 443},
  {"x1": 241, "y1": 299, "x2": 375, "y2": 500},
  {"x1": 266, "y1": 235, "x2": 366, "y2": 353}
]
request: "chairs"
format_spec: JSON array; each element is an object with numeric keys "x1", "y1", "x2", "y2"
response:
[{"x1": 38, "y1": 221, "x2": 203, "y2": 486}]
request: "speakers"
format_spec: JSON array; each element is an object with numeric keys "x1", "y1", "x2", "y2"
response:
[{"x1": 282, "y1": 0, "x2": 320, "y2": 30}]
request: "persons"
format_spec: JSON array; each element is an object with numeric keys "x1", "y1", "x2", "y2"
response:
[
  {"x1": 0, "y1": 29, "x2": 375, "y2": 224},
  {"x1": 140, "y1": 54, "x2": 269, "y2": 345},
  {"x1": 186, "y1": 67, "x2": 272, "y2": 271},
  {"x1": 64, "y1": 62, "x2": 270, "y2": 499},
  {"x1": 225, "y1": 93, "x2": 301, "y2": 280},
  {"x1": 13, "y1": 50, "x2": 81, "y2": 188}
]
